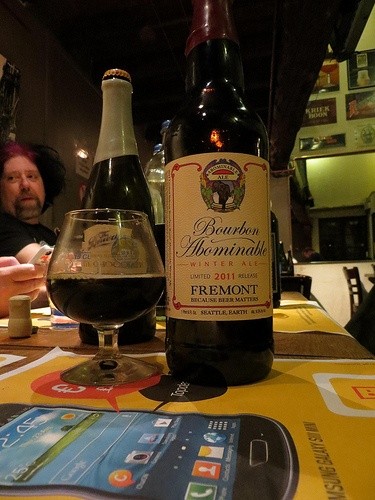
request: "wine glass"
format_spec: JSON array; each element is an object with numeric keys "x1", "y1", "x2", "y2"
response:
[{"x1": 44, "y1": 208, "x2": 165, "y2": 386}]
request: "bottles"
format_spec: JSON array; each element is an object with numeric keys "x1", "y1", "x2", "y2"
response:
[
  {"x1": 77, "y1": 68, "x2": 156, "y2": 347},
  {"x1": 279, "y1": 240, "x2": 294, "y2": 277},
  {"x1": 160, "y1": 0, "x2": 279, "y2": 387}
]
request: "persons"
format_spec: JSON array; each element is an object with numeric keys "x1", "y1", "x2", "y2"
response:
[
  {"x1": 0, "y1": 140, "x2": 66, "y2": 309},
  {"x1": 0, "y1": 255, "x2": 50, "y2": 318}
]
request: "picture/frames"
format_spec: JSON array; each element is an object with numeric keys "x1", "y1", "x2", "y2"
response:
[{"x1": 347, "y1": 49, "x2": 375, "y2": 91}]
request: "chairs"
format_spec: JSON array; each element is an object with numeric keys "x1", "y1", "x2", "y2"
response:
[{"x1": 343, "y1": 266, "x2": 365, "y2": 320}]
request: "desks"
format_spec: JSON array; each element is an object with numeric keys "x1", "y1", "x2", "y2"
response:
[
  {"x1": 278, "y1": 274, "x2": 312, "y2": 300},
  {"x1": 0, "y1": 292, "x2": 374, "y2": 500}
]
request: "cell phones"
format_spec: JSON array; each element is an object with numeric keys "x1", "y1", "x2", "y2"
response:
[
  {"x1": 27, "y1": 245, "x2": 53, "y2": 265},
  {"x1": 0, "y1": 403, "x2": 301, "y2": 500}
]
